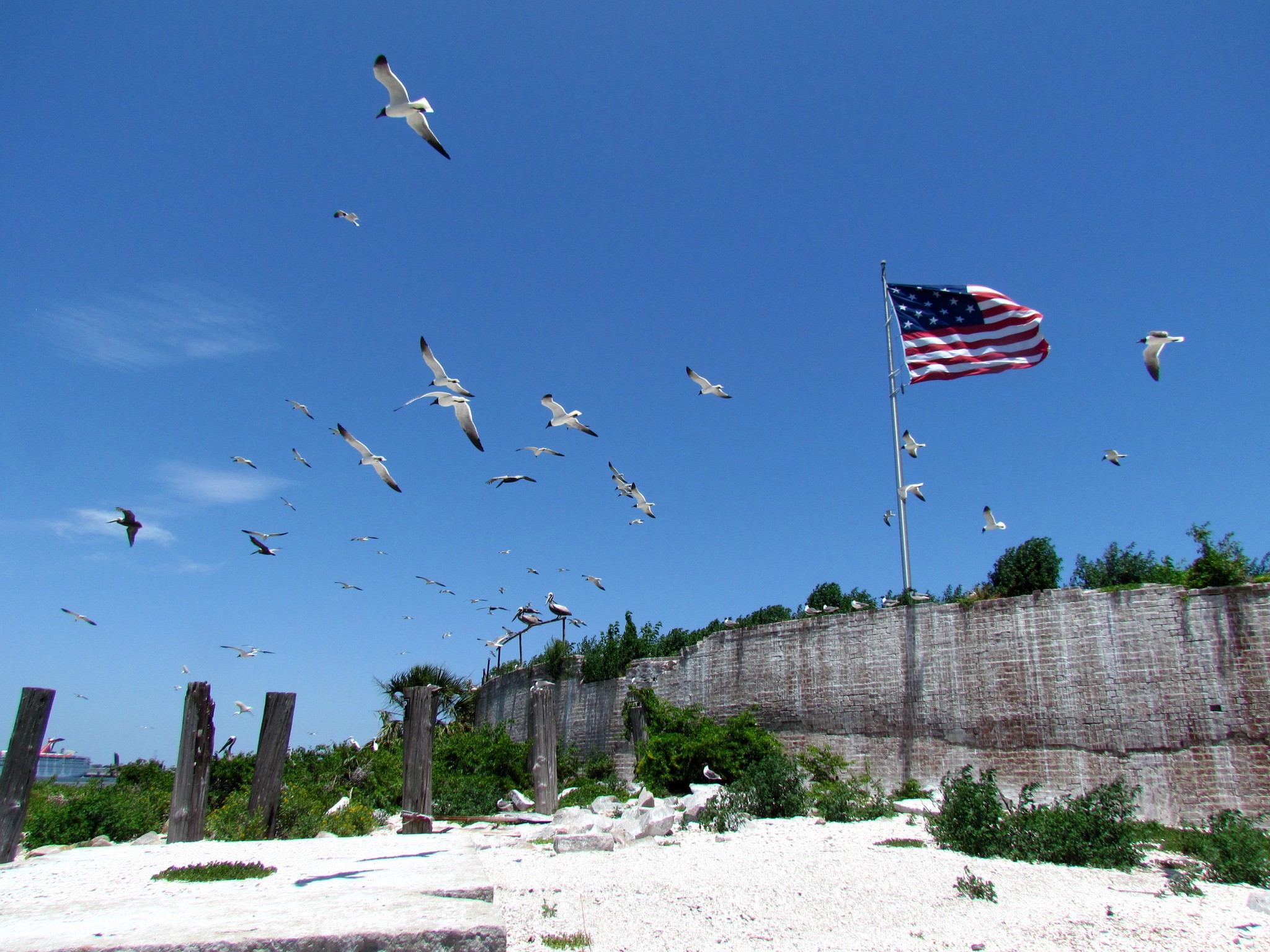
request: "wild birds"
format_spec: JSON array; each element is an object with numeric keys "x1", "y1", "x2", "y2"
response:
[
  {"x1": 219, "y1": 736, "x2": 237, "y2": 761},
  {"x1": 136, "y1": 725, "x2": 154, "y2": 729},
  {"x1": 233, "y1": 701, "x2": 255, "y2": 716},
  {"x1": 334, "y1": 581, "x2": 364, "y2": 591},
  {"x1": 882, "y1": 328, "x2": 1184, "y2": 535},
  {"x1": 418, "y1": 336, "x2": 475, "y2": 397},
  {"x1": 182, "y1": 663, "x2": 191, "y2": 675},
  {"x1": 701, "y1": 762, "x2": 722, "y2": 784},
  {"x1": 373, "y1": 54, "x2": 452, "y2": 159},
  {"x1": 497, "y1": 800, "x2": 513, "y2": 812},
  {"x1": 250, "y1": 535, "x2": 283, "y2": 556},
  {"x1": 371, "y1": 737, "x2": 378, "y2": 751},
  {"x1": 804, "y1": 588, "x2": 929, "y2": 616},
  {"x1": 723, "y1": 617, "x2": 739, "y2": 626},
  {"x1": 334, "y1": 211, "x2": 361, "y2": 228},
  {"x1": 174, "y1": 685, "x2": 183, "y2": 691},
  {"x1": 220, "y1": 644, "x2": 276, "y2": 658},
  {"x1": 345, "y1": 736, "x2": 361, "y2": 749},
  {"x1": 62, "y1": 608, "x2": 97, "y2": 625},
  {"x1": 105, "y1": 506, "x2": 143, "y2": 548},
  {"x1": 231, "y1": 365, "x2": 732, "y2": 690},
  {"x1": 74, "y1": 694, "x2": 89, "y2": 699},
  {"x1": 241, "y1": 530, "x2": 289, "y2": 540}
]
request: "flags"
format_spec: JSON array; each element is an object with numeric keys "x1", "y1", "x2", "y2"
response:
[{"x1": 886, "y1": 282, "x2": 1050, "y2": 385}]
